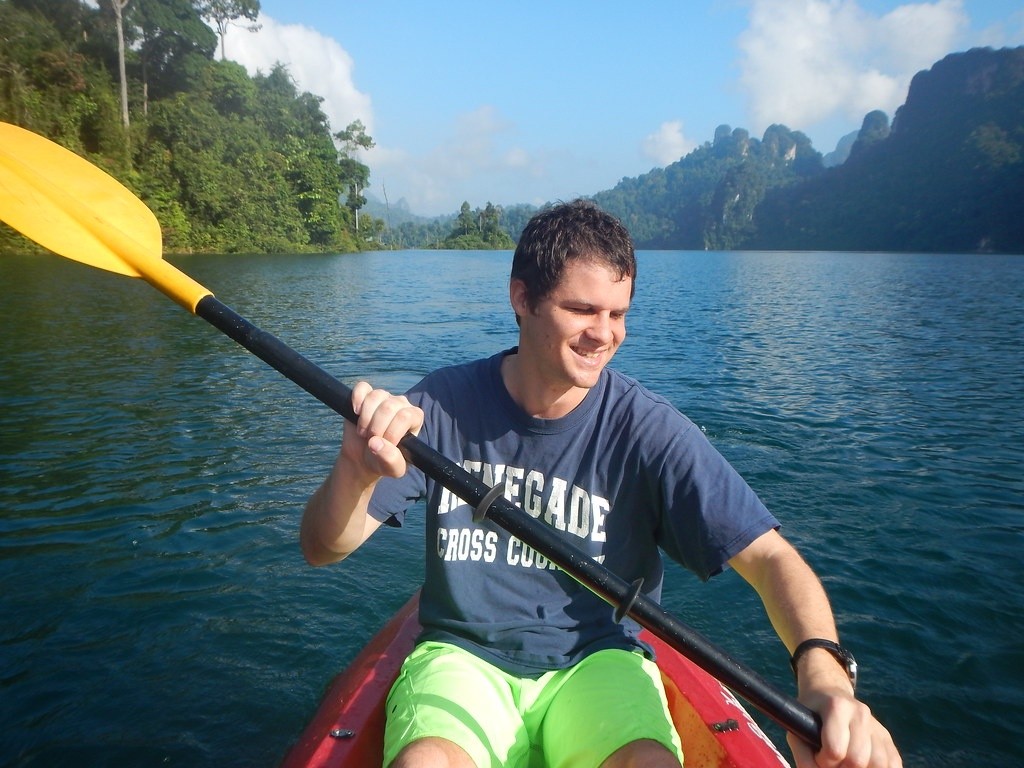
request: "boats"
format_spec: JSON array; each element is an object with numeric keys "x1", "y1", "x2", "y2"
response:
[{"x1": 283, "y1": 564, "x2": 790, "y2": 768}]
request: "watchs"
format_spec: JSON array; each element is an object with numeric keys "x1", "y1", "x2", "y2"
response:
[{"x1": 791, "y1": 638, "x2": 858, "y2": 690}]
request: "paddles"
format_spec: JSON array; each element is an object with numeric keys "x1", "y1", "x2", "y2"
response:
[{"x1": 1, "y1": 117, "x2": 826, "y2": 751}]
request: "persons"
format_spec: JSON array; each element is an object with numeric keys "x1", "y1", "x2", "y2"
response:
[{"x1": 299, "y1": 203, "x2": 903, "y2": 768}]
